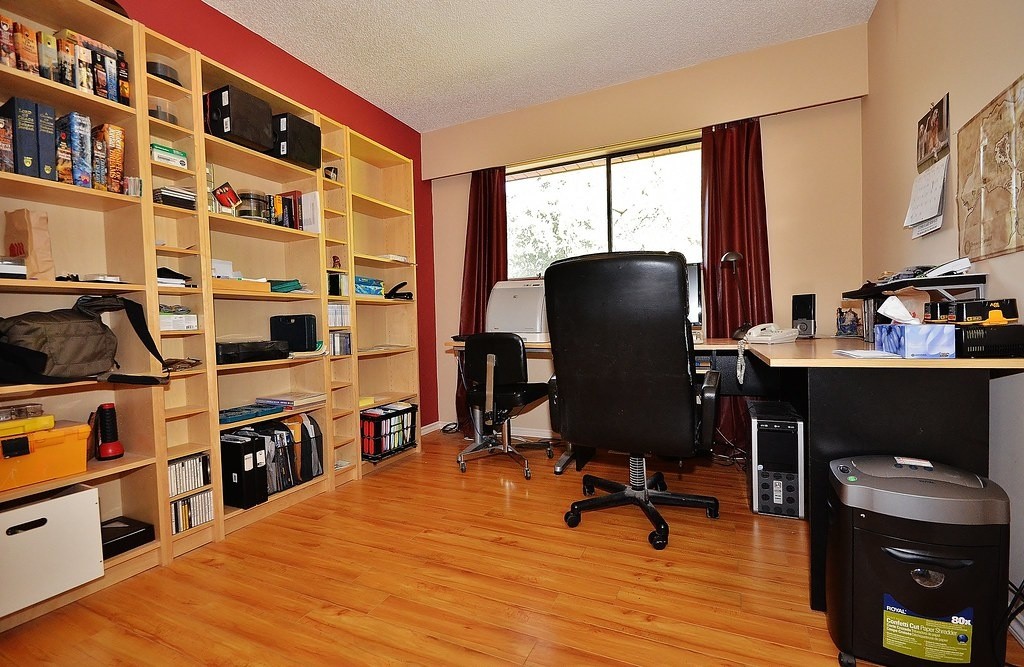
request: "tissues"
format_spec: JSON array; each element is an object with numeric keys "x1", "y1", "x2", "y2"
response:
[{"x1": 874, "y1": 296, "x2": 956, "y2": 359}]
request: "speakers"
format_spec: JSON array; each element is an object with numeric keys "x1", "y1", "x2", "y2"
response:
[
  {"x1": 203, "y1": 84, "x2": 321, "y2": 172},
  {"x1": 791, "y1": 293, "x2": 816, "y2": 334}
]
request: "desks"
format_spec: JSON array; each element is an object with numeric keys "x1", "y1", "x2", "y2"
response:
[
  {"x1": 748, "y1": 338, "x2": 1024, "y2": 613},
  {"x1": 443, "y1": 334, "x2": 831, "y2": 476}
]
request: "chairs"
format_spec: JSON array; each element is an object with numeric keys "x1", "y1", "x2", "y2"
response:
[
  {"x1": 544, "y1": 251, "x2": 722, "y2": 549},
  {"x1": 456, "y1": 332, "x2": 549, "y2": 480}
]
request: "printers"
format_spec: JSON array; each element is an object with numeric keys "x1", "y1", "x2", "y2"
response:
[{"x1": 485, "y1": 279, "x2": 552, "y2": 341}]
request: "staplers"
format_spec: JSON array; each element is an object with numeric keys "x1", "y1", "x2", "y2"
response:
[{"x1": 385, "y1": 281, "x2": 413, "y2": 299}]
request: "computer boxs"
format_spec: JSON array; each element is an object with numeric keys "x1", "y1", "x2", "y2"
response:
[{"x1": 742, "y1": 400, "x2": 807, "y2": 521}]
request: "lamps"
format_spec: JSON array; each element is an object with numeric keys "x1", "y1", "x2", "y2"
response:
[{"x1": 721, "y1": 251, "x2": 752, "y2": 341}]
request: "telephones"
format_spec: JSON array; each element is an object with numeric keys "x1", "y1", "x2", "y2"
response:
[{"x1": 743, "y1": 322, "x2": 799, "y2": 345}]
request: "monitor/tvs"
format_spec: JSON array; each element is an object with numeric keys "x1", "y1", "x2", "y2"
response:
[{"x1": 687, "y1": 263, "x2": 703, "y2": 327}]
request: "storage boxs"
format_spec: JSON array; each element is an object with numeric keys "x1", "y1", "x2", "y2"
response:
[
  {"x1": 0, "y1": 483, "x2": 104, "y2": 618},
  {"x1": 150, "y1": 143, "x2": 188, "y2": 169},
  {"x1": 212, "y1": 278, "x2": 271, "y2": 293},
  {"x1": 159, "y1": 314, "x2": 198, "y2": 331},
  {"x1": 360, "y1": 404, "x2": 419, "y2": 465},
  {"x1": 0, "y1": 420, "x2": 91, "y2": 492}
]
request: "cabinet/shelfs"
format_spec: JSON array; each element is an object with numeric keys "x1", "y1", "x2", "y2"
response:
[
  {"x1": 139, "y1": 22, "x2": 221, "y2": 564},
  {"x1": 0, "y1": 0, "x2": 168, "y2": 634},
  {"x1": 317, "y1": 112, "x2": 361, "y2": 490},
  {"x1": 195, "y1": 49, "x2": 333, "y2": 542},
  {"x1": 346, "y1": 126, "x2": 422, "y2": 479}
]
